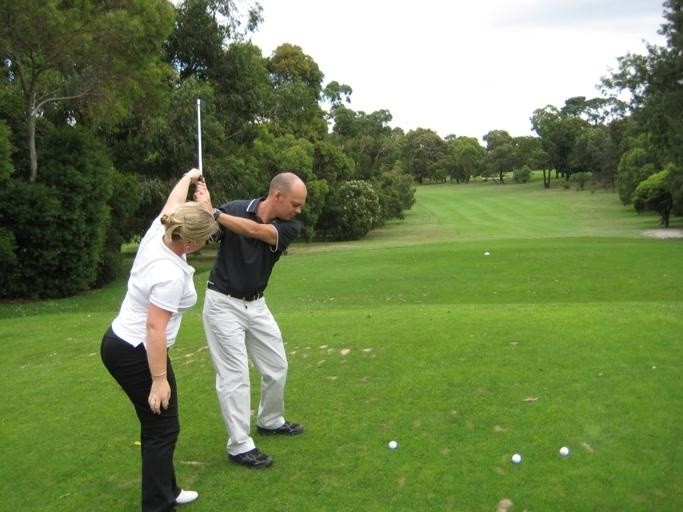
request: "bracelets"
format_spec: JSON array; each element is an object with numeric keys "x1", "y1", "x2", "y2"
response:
[
  {"x1": 149, "y1": 371, "x2": 167, "y2": 379},
  {"x1": 182, "y1": 173, "x2": 193, "y2": 184},
  {"x1": 212, "y1": 208, "x2": 220, "y2": 223}
]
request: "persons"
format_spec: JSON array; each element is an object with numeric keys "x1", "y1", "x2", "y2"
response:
[
  {"x1": 191, "y1": 170, "x2": 310, "y2": 470},
  {"x1": 100, "y1": 167, "x2": 222, "y2": 511}
]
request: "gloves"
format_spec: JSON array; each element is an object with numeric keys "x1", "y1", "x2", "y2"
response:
[{"x1": 184, "y1": 167, "x2": 205, "y2": 184}]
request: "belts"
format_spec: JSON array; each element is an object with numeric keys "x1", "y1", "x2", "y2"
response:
[{"x1": 208, "y1": 283, "x2": 264, "y2": 300}]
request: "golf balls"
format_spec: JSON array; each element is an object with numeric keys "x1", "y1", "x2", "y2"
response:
[
  {"x1": 388, "y1": 441, "x2": 398, "y2": 450},
  {"x1": 511, "y1": 454, "x2": 522, "y2": 464},
  {"x1": 559, "y1": 446, "x2": 570, "y2": 458}
]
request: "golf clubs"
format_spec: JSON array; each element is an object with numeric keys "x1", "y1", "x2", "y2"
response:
[{"x1": 195, "y1": 89, "x2": 205, "y2": 182}]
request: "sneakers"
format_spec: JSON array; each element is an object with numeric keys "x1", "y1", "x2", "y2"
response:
[
  {"x1": 257, "y1": 418, "x2": 304, "y2": 434},
  {"x1": 176, "y1": 490, "x2": 198, "y2": 504},
  {"x1": 228, "y1": 449, "x2": 273, "y2": 469}
]
